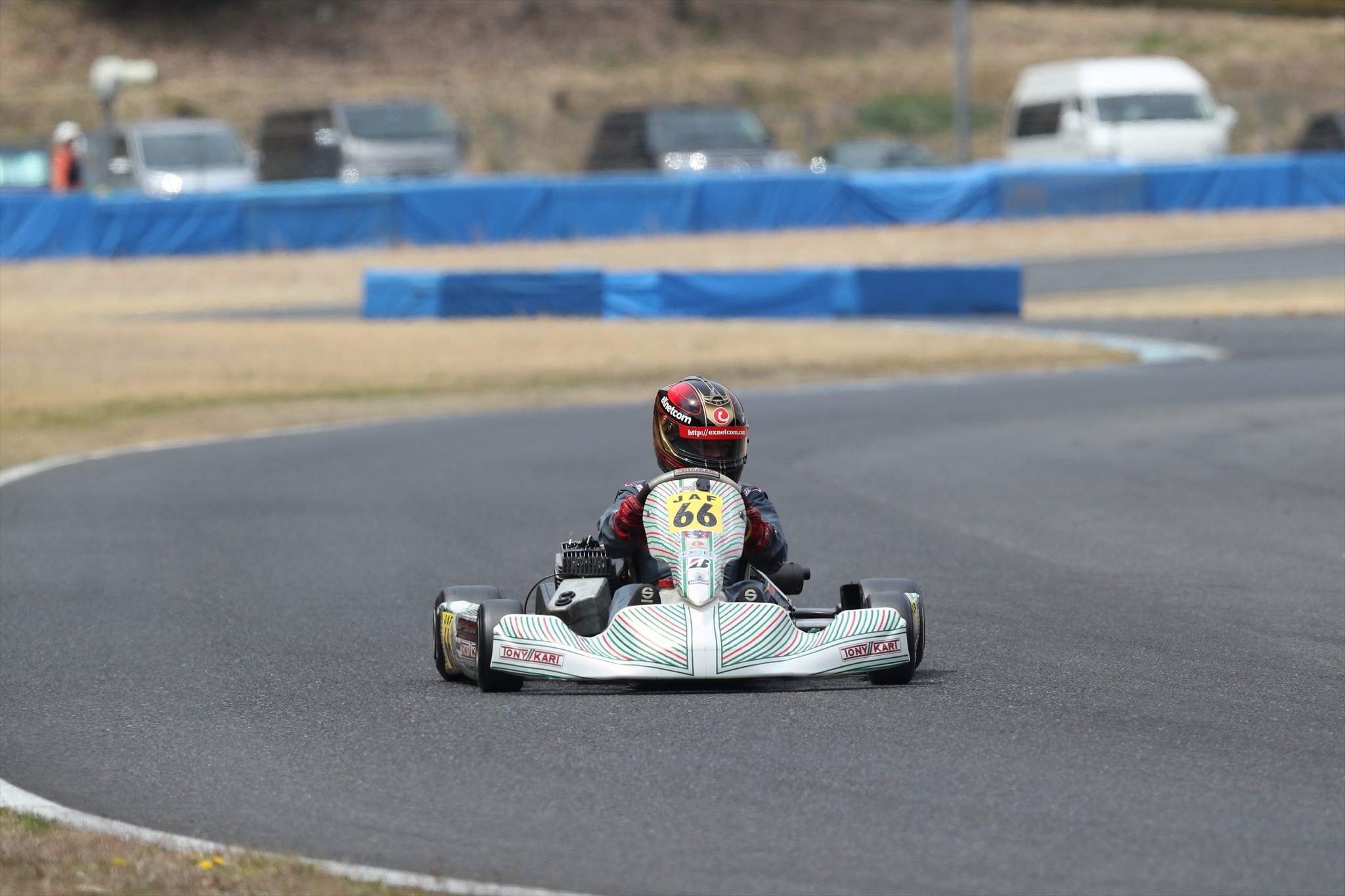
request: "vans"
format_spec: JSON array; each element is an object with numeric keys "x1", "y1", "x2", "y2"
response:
[
  {"x1": 260, "y1": 96, "x2": 468, "y2": 183},
  {"x1": 83, "y1": 116, "x2": 265, "y2": 197},
  {"x1": 1005, "y1": 53, "x2": 1240, "y2": 164},
  {"x1": 588, "y1": 102, "x2": 790, "y2": 177}
]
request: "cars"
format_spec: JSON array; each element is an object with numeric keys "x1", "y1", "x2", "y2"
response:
[
  {"x1": 1287, "y1": 110, "x2": 1345, "y2": 147},
  {"x1": 811, "y1": 135, "x2": 940, "y2": 172}
]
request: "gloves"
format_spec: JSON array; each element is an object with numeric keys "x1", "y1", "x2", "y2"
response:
[
  {"x1": 611, "y1": 494, "x2": 644, "y2": 539},
  {"x1": 743, "y1": 505, "x2": 771, "y2": 554}
]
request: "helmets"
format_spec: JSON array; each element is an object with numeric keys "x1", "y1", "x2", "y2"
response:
[{"x1": 652, "y1": 376, "x2": 749, "y2": 483}]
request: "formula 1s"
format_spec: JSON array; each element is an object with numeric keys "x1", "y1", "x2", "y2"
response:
[{"x1": 430, "y1": 466, "x2": 927, "y2": 694}]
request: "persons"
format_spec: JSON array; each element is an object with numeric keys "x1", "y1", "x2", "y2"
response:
[
  {"x1": 50, "y1": 118, "x2": 84, "y2": 192},
  {"x1": 596, "y1": 377, "x2": 789, "y2": 607}
]
